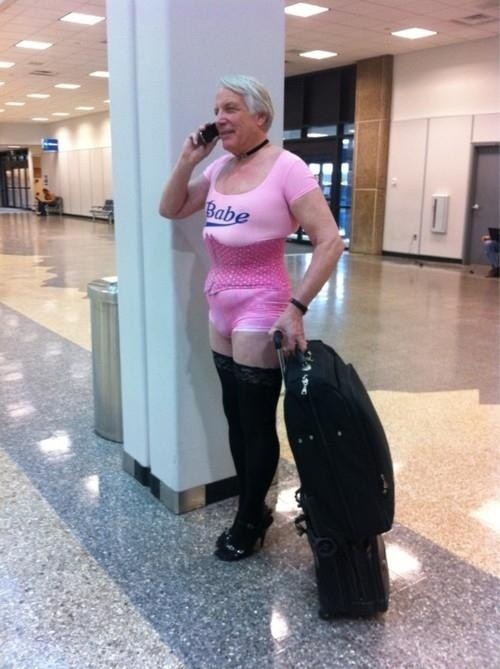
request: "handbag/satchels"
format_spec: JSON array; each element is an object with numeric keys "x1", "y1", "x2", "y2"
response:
[{"x1": 282, "y1": 340, "x2": 394, "y2": 538}]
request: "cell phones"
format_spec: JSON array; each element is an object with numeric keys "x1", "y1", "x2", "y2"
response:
[{"x1": 199, "y1": 122, "x2": 219, "y2": 145}]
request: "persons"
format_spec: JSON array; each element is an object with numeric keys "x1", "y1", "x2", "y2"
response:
[
  {"x1": 36, "y1": 188, "x2": 57, "y2": 216},
  {"x1": 158, "y1": 74, "x2": 345, "y2": 560},
  {"x1": 481, "y1": 226, "x2": 500, "y2": 278}
]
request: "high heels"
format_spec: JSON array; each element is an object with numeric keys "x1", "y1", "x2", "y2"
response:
[{"x1": 215, "y1": 513, "x2": 274, "y2": 561}]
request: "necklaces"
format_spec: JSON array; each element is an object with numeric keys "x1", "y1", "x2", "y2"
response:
[{"x1": 236, "y1": 138, "x2": 270, "y2": 160}]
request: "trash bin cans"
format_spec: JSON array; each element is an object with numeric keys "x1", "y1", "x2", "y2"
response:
[{"x1": 87, "y1": 276, "x2": 122, "y2": 443}]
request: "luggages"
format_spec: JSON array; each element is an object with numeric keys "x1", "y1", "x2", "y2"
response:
[{"x1": 274, "y1": 332, "x2": 396, "y2": 618}]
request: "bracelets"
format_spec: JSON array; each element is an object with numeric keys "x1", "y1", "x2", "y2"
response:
[{"x1": 289, "y1": 298, "x2": 308, "y2": 316}]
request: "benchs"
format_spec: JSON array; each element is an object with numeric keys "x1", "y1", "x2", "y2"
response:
[
  {"x1": 89, "y1": 200, "x2": 114, "y2": 225},
  {"x1": 44, "y1": 196, "x2": 63, "y2": 216}
]
